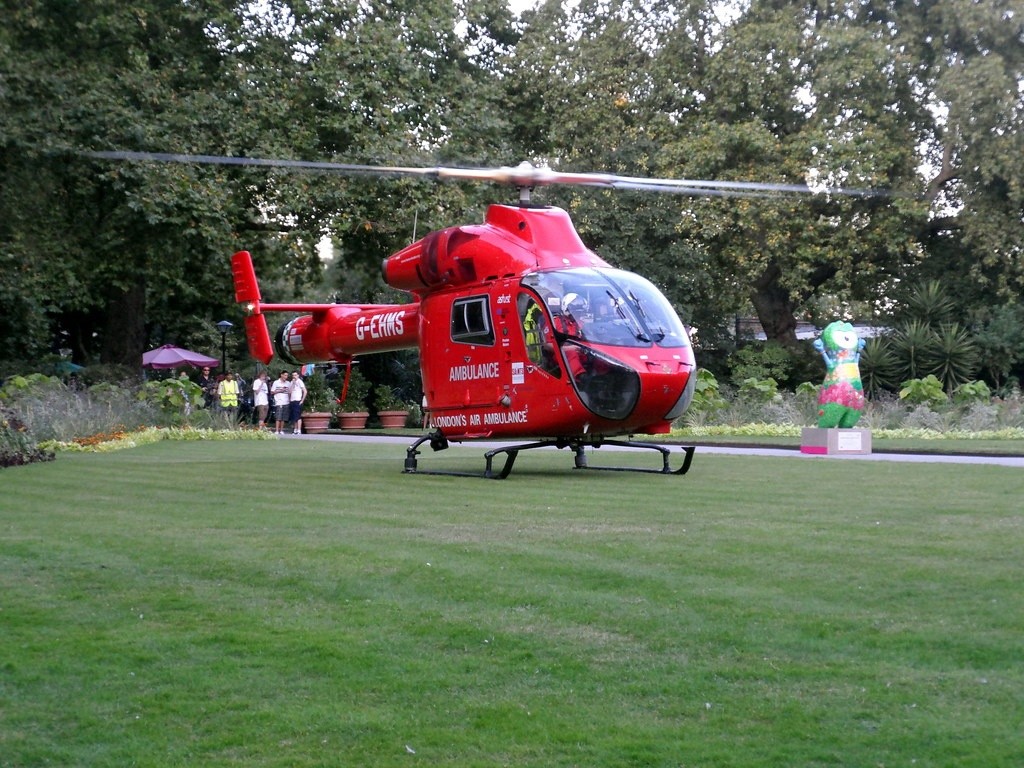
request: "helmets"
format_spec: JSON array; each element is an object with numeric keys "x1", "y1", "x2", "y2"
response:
[{"x1": 561, "y1": 293, "x2": 589, "y2": 316}]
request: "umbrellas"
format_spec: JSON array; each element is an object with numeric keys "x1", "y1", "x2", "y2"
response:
[{"x1": 142, "y1": 345, "x2": 219, "y2": 369}]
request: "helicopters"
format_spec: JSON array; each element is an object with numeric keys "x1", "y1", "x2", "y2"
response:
[{"x1": 63, "y1": 147, "x2": 882, "y2": 478}]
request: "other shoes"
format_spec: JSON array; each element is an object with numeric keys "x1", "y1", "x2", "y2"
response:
[
  {"x1": 281, "y1": 431, "x2": 284, "y2": 435},
  {"x1": 275, "y1": 431, "x2": 279, "y2": 435},
  {"x1": 292, "y1": 431, "x2": 300, "y2": 435}
]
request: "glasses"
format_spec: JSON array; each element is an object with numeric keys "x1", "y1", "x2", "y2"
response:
[{"x1": 203, "y1": 369, "x2": 210, "y2": 372}]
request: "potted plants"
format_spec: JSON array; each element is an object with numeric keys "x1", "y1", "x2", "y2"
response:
[
  {"x1": 335, "y1": 366, "x2": 372, "y2": 429},
  {"x1": 373, "y1": 384, "x2": 410, "y2": 429},
  {"x1": 300, "y1": 366, "x2": 334, "y2": 434}
]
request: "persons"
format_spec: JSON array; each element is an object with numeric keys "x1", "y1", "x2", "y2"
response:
[
  {"x1": 541, "y1": 293, "x2": 609, "y2": 395},
  {"x1": 178, "y1": 366, "x2": 307, "y2": 435},
  {"x1": 422, "y1": 395, "x2": 434, "y2": 429}
]
